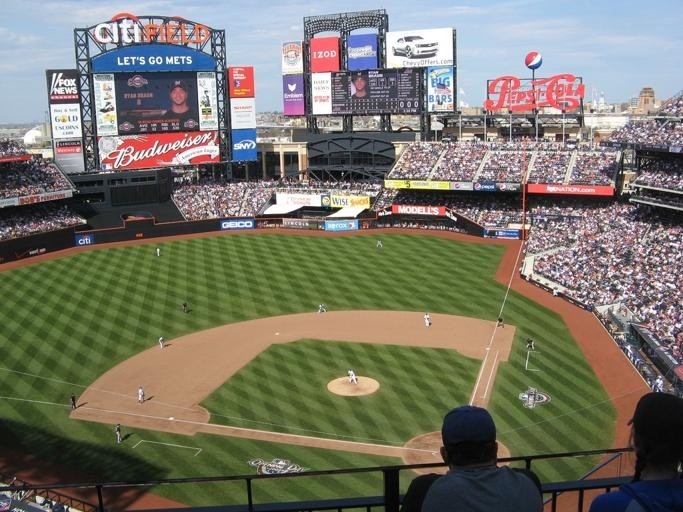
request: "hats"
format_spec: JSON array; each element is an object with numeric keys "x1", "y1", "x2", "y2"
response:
[
  {"x1": 627, "y1": 393, "x2": 682, "y2": 426},
  {"x1": 441, "y1": 406, "x2": 495, "y2": 446}
]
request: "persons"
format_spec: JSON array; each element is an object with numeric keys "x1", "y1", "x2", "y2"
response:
[
  {"x1": 159, "y1": 335, "x2": 165, "y2": 349},
  {"x1": 319, "y1": 304, "x2": 327, "y2": 313},
  {"x1": 610, "y1": 90, "x2": 683, "y2": 210},
  {"x1": 400, "y1": 405, "x2": 545, "y2": 512},
  {"x1": 171, "y1": 176, "x2": 402, "y2": 228},
  {"x1": 0, "y1": 135, "x2": 87, "y2": 242},
  {"x1": 156, "y1": 247, "x2": 160, "y2": 257},
  {"x1": 8, "y1": 477, "x2": 69, "y2": 512},
  {"x1": 183, "y1": 302, "x2": 188, "y2": 313},
  {"x1": 386, "y1": 139, "x2": 624, "y2": 236},
  {"x1": 497, "y1": 317, "x2": 505, "y2": 328},
  {"x1": 424, "y1": 312, "x2": 433, "y2": 327},
  {"x1": 161, "y1": 79, "x2": 199, "y2": 131},
  {"x1": 116, "y1": 424, "x2": 122, "y2": 444},
  {"x1": 588, "y1": 391, "x2": 683, "y2": 512},
  {"x1": 138, "y1": 386, "x2": 144, "y2": 404},
  {"x1": 527, "y1": 213, "x2": 683, "y2": 397},
  {"x1": 350, "y1": 71, "x2": 370, "y2": 99},
  {"x1": 71, "y1": 392, "x2": 76, "y2": 410},
  {"x1": 348, "y1": 369, "x2": 359, "y2": 385}
]
